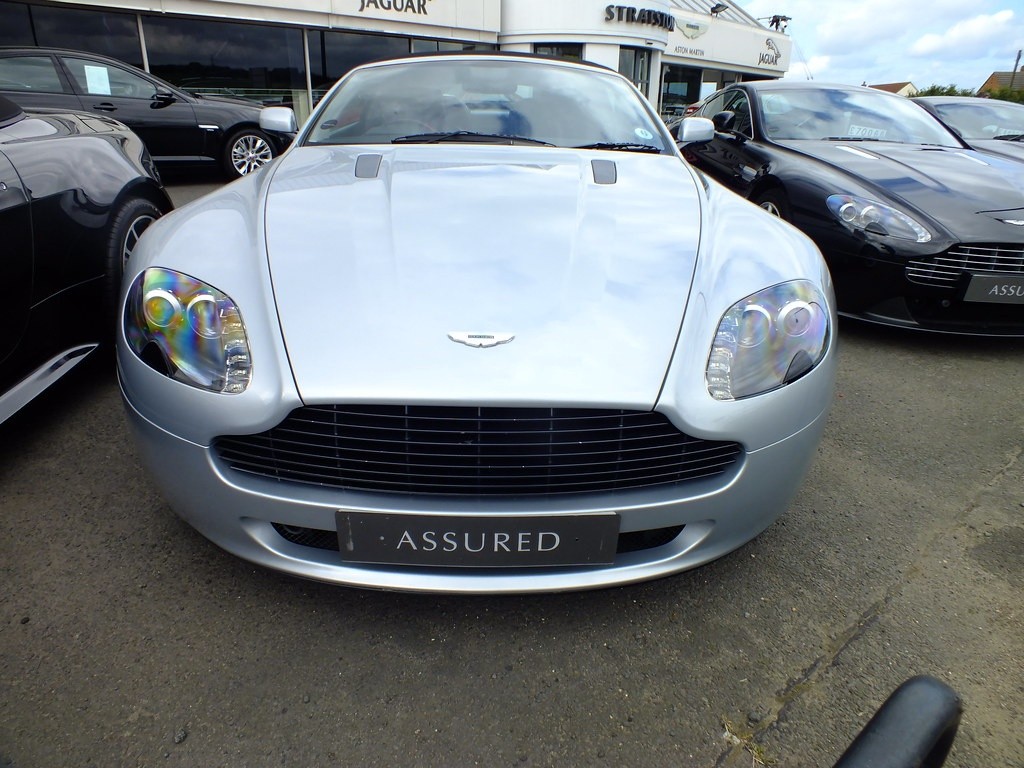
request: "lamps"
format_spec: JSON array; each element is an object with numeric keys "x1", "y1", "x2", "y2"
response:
[{"x1": 711, "y1": 3, "x2": 727, "y2": 16}]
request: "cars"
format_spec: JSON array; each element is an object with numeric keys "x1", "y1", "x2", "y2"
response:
[
  {"x1": 1, "y1": 45, "x2": 298, "y2": 183},
  {"x1": 668, "y1": 82, "x2": 1024, "y2": 337},
  {"x1": 847, "y1": 96, "x2": 1024, "y2": 164},
  {"x1": 118, "y1": 50, "x2": 839, "y2": 594},
  {"x1": 1, "y1": 94, "x2": 177, "y2": 425}
]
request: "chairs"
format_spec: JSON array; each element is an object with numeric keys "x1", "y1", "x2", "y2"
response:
[{"x1": 440, "y1": 93, "x2": 472, "y2": 133}]
